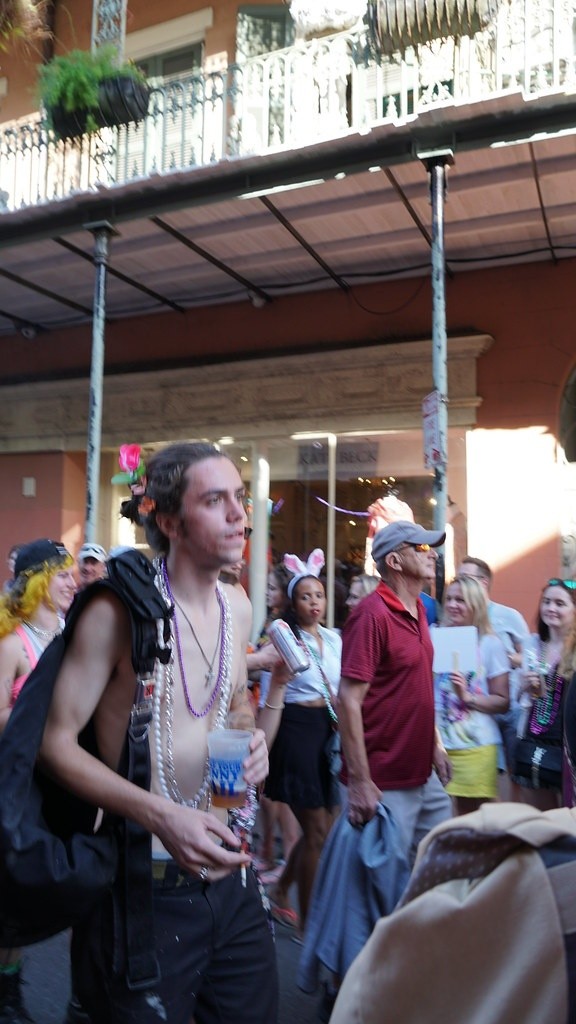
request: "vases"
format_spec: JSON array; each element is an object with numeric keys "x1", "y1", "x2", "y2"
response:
[{"x1": 365, "y1": 0, "x2": 495, "y2": 53}]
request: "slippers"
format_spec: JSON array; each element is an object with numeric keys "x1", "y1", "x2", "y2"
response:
[{"x1": 269, "y1": 906, "x2": 300, "y2": 928}]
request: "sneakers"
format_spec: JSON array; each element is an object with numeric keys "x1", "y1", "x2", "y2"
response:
[{"x1": 0, "y1": 956, "x2": 36, "y2": 1024}]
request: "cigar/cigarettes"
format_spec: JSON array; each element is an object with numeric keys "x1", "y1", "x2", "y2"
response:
[{"x1": 240, "y1": 850, "x2": 246, "y2": 888}]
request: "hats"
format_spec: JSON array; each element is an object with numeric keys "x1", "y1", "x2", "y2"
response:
[
  {"x1": 79, "y1": 543, "x2": 108, "y2": 564},
  {"x1": 371, "y1": 521, "x2": 446, "y2": 562},
  {"x1": 14, "y1": 539, "x2": 69, "y2": 579}
]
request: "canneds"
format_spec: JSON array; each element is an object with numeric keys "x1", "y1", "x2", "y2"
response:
[{"x1": 266, "y1": 618, "x2": 310, "y2": 674}]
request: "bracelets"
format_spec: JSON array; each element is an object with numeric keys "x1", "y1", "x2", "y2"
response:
[{"x1": 265, "y1": 703, "x2": 284, "y2": 709}]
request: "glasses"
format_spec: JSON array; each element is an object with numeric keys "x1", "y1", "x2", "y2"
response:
[
  {"x1": 547, "y1": 578, "x2": 576, "y2": 589},
  {"x1": 395, "y1": 544, "x2": 430, "y2": 552}
]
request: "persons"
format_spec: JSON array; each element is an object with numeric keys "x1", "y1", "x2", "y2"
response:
[
  {"x1": 517, "y1": 576, "x2": 576, "y2": 812},
  {"x1": 434, "y1": 576, "x2": 509, "y2": 814},
  {"x1": 365, "y1": 497, "x2": 438, "y2": 624},
  {"x1": 40, "y1": 444, "x2": 277, "y2": 1023},
  {"x1": 79, "y1": 542, "x2": 107, "y2": 582},
  {"x1": 247, "y1": 547, "x2": 343, "y2": 944},
  {"x1": 345, "y1": 574, "x2": 380, "y2": 610},
  {"x1": 338, "y1": 520, "x2": 453, "y2": 859},
  {"x1": 0, "y1": 540, "x2": 86, "y2": 1024},
  {"x1": 460, "y1": 557, "x2": 530, "y2": 802}
]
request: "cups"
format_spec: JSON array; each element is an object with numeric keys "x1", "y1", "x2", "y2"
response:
[{"x1": 207, "y1": 728, "x2": 254, "y2": 809}]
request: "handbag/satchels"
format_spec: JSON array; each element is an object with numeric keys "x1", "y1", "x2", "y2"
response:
[
  {"x1": 0, "y1": 545, "x2": 159, "y2": 988},
  {"x1": 513, "y1": 739, "x2": 563, "y2": 791}
]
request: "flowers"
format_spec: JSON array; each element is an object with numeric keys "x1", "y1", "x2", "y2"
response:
[{"x1": 110, "y1": 443, "x2": 147, "y2": 487}]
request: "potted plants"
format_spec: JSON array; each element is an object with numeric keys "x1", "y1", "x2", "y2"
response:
[{"x1": 29, "y1": 45, "x2": 153, "y2": 144}]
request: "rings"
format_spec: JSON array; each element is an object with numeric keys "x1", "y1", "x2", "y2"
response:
[{"x1": 199, "y1": 867, "x2": 208, "y2": 880}]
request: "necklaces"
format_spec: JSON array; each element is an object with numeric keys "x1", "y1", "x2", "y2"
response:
[
  {"x1": 298, "y1": 626, "x2": 339, "y2": 723},
  {"x1": 154, "y1": 555, "x2": 233, "y2": 810},
  {"x1": 24, "y1": 619, "x2": 62, "y2": 636}
]
